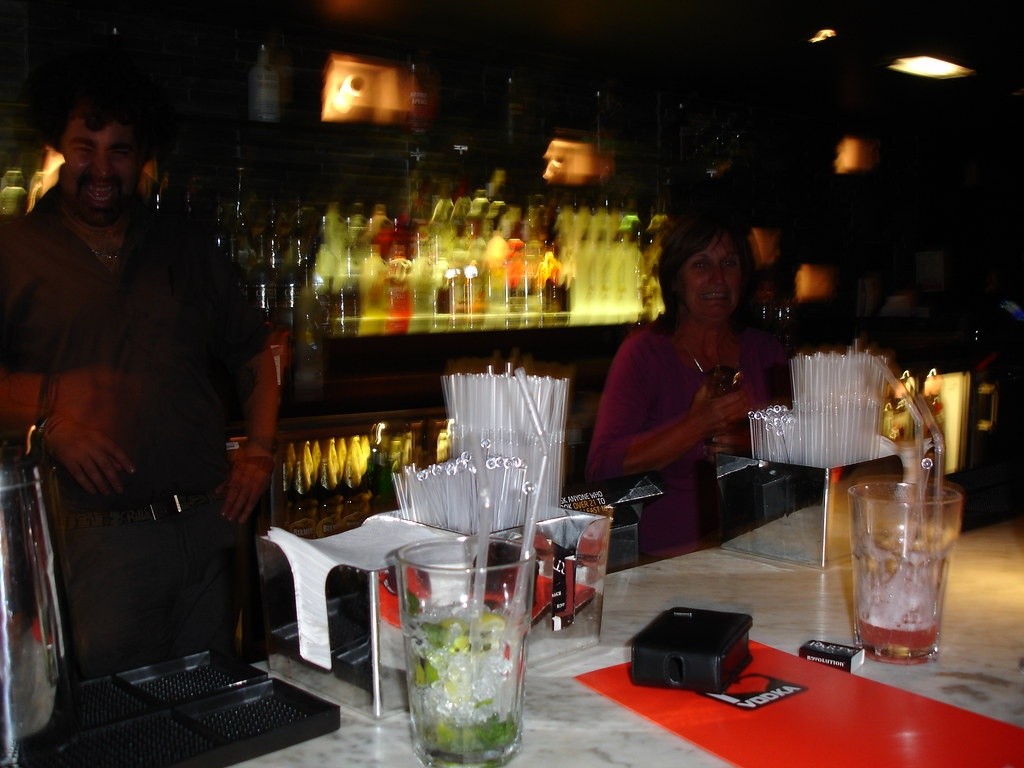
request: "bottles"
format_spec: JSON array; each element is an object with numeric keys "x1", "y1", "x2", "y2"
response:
[
  {"x1": 276, "y1": 418, "x2": 451, "y2": 591},
  {"x1": 186, "y1": 27, "x2": 672, "y2": 344},
  {"x1": 292, "y1": 287, "x2": 325, "y2": 404}
]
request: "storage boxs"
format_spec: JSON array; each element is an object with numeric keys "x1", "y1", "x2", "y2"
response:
[{"x1": 799, "y1": 640, "x2": 865, "y2": 673}]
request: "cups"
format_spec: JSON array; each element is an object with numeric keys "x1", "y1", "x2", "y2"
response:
[
  {"x1": 702, "y1": 358, "x2": 751, "y2": 428},
  {"x1": 848, "y1": 483, "x2": 961, "y2": 664},
  {"x1": 397, "y1": 538, "x2": 537, "y2": 768}
]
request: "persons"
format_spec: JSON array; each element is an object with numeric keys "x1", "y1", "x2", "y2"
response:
[
  {"x1": 585, "y1": 213, "x2": 795, "y2": 563},
  {"x1": 0, "y1": 94, "x2": 290, "y2": 680}
]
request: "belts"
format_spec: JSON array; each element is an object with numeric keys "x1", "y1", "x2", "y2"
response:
[{"x1": 62, "y1": 487, "x2": 227, "y2": 529}]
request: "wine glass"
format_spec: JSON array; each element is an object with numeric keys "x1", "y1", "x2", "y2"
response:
[{"x1": 756, "y1": 299, "x2": 796, "y2": 352}]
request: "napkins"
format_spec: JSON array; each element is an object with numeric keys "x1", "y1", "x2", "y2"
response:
[{"x1": 268, "y1": 516, "x2": 475, "y2": 670}]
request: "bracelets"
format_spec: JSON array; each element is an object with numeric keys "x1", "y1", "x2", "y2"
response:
[{"x1": 35, "y1": 410, "x2": 65, "y2": 449}]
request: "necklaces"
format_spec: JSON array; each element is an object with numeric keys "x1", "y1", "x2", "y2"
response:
[{"x1": 92, "y1": 248, "x2": 119, "y2": 260}]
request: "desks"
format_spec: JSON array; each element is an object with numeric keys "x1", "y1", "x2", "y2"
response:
[{"x1": 237, "y1": 513, "x2": 1024, "y2": 768}]
